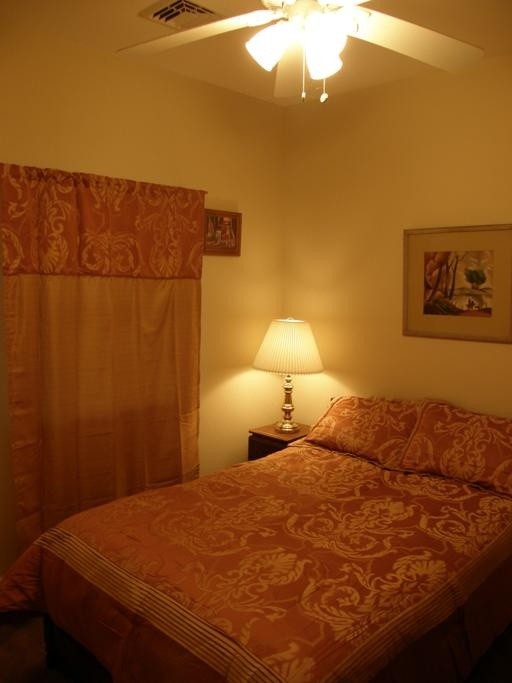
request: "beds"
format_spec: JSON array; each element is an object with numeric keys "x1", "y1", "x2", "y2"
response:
[{"x1": 43, "y1": 421, "x2": 511, "y2": 677}]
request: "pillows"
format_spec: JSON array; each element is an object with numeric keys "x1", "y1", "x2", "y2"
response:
[
  {"x1": 308, "y1": 392, "x2": 414, "y2": 470},
  {"x1": 405, "y1": 397, "x2": 510, "y2": 493}
]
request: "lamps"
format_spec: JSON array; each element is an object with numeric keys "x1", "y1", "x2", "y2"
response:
[
  {"x1": 255, "y1": 316, "x2": 323, "y2": 431},
  {"x1": 245, "y1": 0, "x2": 350, "y2": 106}
]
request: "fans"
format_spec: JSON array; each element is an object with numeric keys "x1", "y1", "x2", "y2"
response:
[{"x1": 114, "y1": 3, "x2": 486, "y2": 101}]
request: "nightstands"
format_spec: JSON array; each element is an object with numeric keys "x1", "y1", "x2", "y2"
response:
[{"x1": 246, "y1": 422, "x2": 309, "y2": 459}]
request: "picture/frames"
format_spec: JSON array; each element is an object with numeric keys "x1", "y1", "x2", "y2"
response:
[
  {"x1": 204, "y1": 208, "x2": 242, "y2": 257},
  {"x1": 400, "y1": 221, "x2": 509, "y2": 346}
]
